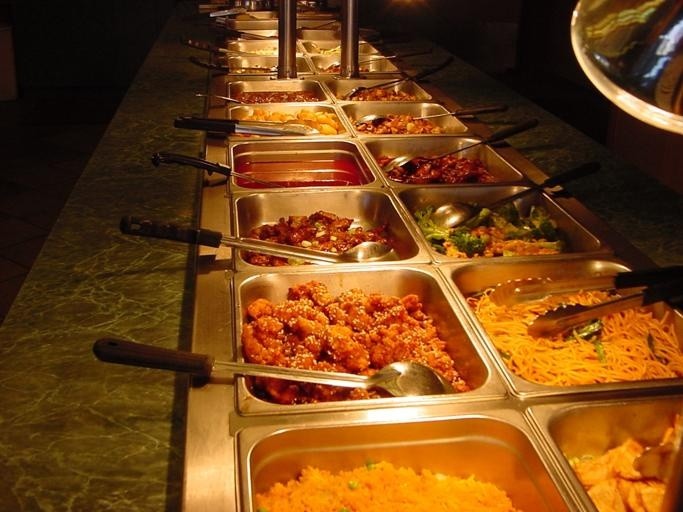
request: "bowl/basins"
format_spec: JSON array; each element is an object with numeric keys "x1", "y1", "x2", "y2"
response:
[
  {"x1": 524, "y1": 389, "x2": 683, "y2": 512},
  {"x1": 320, "y1": 76, "x2": 434, "y2": 104},
  {"x1": 230, "y1": 140, "x2": 384, "y2": 190},
  {"x1": 296, "y1": 12, "x2": 337, "y2": 18},
  {"x1": 229, "y1": 42, "x2": 304, "y2": 57},
  {"x1": 435, "y1": 255, "x2": 683, "y2": 395},
  {"x1": 227, "y1": 11, "x2": 276, "y2": 20},
  {"x1": 305, "y1": 54, "x2": 400, "y2": 75},
  {"x1": 227, "y1": 21, "x2": 278, "y2": 31},
  {"x1": 358, "y1": 134, "x2": 526, "y2": 187},
  {"x1": 231, "y1": 188, "x2": 433, "y2": 270},
  {"x1": 299, "y1": 40, "x2": 380, "y2": 55},
  {"x1": 571, "y1": 0, "x2": 683, "y2": 137},
  {"x1": 337, "y1": 100, "x2": 469, "y2": 137},
  {"x1": 227, "y1": 31, "x2": 279, "y2": 41},
  {"x1": 228, "y1": 58, "x2": 315, "y2": 75},
  {"x1": 232, "y1": 266, "x2": 509, "y2": 417},
  {"x1": 296, "y1": 19, "x2": 341, "y2": 29},
  {"x1": 390, "y1": 180, "x2": 602, "y2": 265},
  {"x1": 226, "y1": 80, "x2": 334, "y2": 106},
  {"x1": 295, "y1": 29, "x2": 342, "y2": 41},
  {"x1": 237, "y1": 408, "x2": 582, "y2": 512},
  {"x1": 228, "y1": 103, "x2": 353, "y2": 142}
]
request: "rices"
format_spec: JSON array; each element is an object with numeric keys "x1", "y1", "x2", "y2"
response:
[{"x1": 255, "y1": 463, "x2": 516, "y2": 512}]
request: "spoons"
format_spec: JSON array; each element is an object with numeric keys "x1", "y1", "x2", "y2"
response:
[
  {"x1": 343, "y1": 57, "x2": 453, "y2": 97},
  {"x1": 182, "y1": 37, "x2": 257, "y2": 55},
  {"x1": 356, "y1": 102, "x2": 507, "y2": 126},
  {"x1": 94, "y1": 339, "x2": 459, "y2": 397},
  {"x1": 151, "y1": 150, "x2": 280, "y2": 189},
  {"x1": 120, "y1": 214, "x2": 393, "y2": 263},
  {"x1": 382, "y1": 118, "x2": 541, "y2": 174},
  {"x1": 192, "y1": 90, "x2": 242, "y2": 103},
  {"x1": 188, "y1": 55, "x2": 279, "y2": 72},
  {"x1": 431, "y1": 161, "x2": 600, "y2": 229}
]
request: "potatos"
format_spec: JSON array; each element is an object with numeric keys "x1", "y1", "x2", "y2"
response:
[{"x1": 466, "y1": 288, "x2": 683, "y2": 387}]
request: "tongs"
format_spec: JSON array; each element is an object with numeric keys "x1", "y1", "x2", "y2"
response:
[
  {"x1": 174, "y1": 115, "x2": 319, "y2": 137},
  {"x1": 489, "y1": 262, "x2": 682, "y2": 339}
]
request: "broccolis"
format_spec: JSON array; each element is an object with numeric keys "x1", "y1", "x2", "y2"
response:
[
  {"x1": 452, "y1": 230, "x2": 489, "y2": 256},
  {"x1": 491, "y1": 199, "x2": 558, "y2": 240},
  {"x1": 412, "y1": 203, "x2": 452, "y2": 242},
  {"x1": 526, "y1": 240, "x2": 566, "y2": 252},
  {"x1": 454, "y1": 207, "x2": 491, "y2": 229}
]
